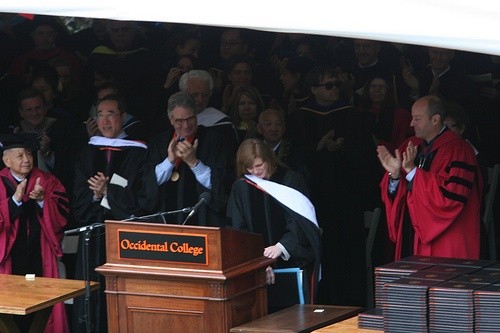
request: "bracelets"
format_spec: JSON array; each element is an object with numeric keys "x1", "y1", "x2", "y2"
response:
[{"x1": 389, "y1": 173, "x2": 400, "y2": 179}]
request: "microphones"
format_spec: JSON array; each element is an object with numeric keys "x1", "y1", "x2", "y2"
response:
[{"x1": 183, "y1": 191, "x2": 213, "y2": 224}]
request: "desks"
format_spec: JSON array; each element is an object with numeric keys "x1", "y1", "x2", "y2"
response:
[
  {"x1": 0, "y1": 273, "x2": 100, "y2": 333},
  {"x1": 229, "y1": 304, "x2": 385, "y2": 333}
]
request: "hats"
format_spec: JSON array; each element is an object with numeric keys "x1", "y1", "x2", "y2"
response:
[{"x1": 3, "y1": 135, "x2": 32, "y2": 150}]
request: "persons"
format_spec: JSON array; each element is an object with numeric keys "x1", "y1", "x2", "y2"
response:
[{"x1": 0, "y1": 13, "x2": 500, "y2": 333}]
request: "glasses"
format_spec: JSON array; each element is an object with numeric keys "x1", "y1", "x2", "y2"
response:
[
  {"x1": 97, "y1": 113, "x2": 123, "y2": 119},
  {"x1": 171, "y1": 115, "x2": 197, "y2": 124},
  {"x1": 317, "y1": 80, "x2": 344, "y2": 90}
]
